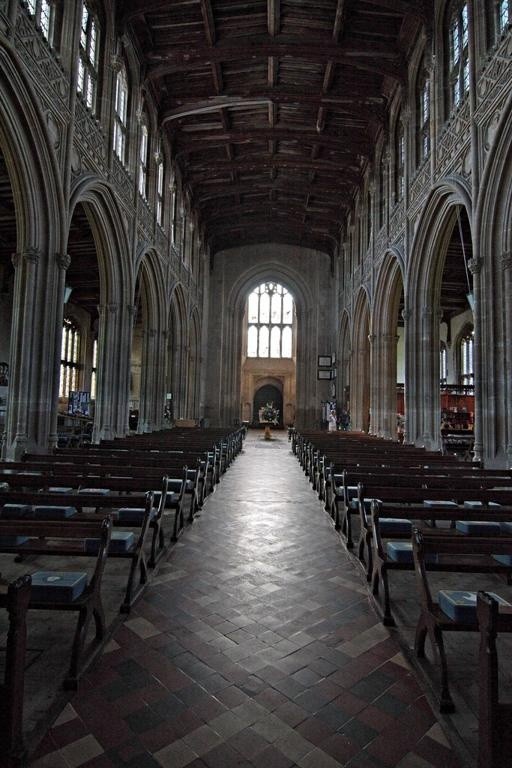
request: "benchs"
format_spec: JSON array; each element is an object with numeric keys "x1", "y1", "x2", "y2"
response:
[
  {"x1": 290, "y1": 427, "x2": 511, "y2": 764},
  {"x1": 1, "y1": 426, "x2": 247, "y2": 768}
]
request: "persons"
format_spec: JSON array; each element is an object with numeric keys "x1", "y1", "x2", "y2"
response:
[
  {"x1": 444, "y1": 424, "x2": 449, "y2": 430},
  {"x1": 339, "y1": 409, "x2": 349, "y2": 431},
  {"x1": 327, "y1": 409, "x2": 338, "y2": 434}
]
